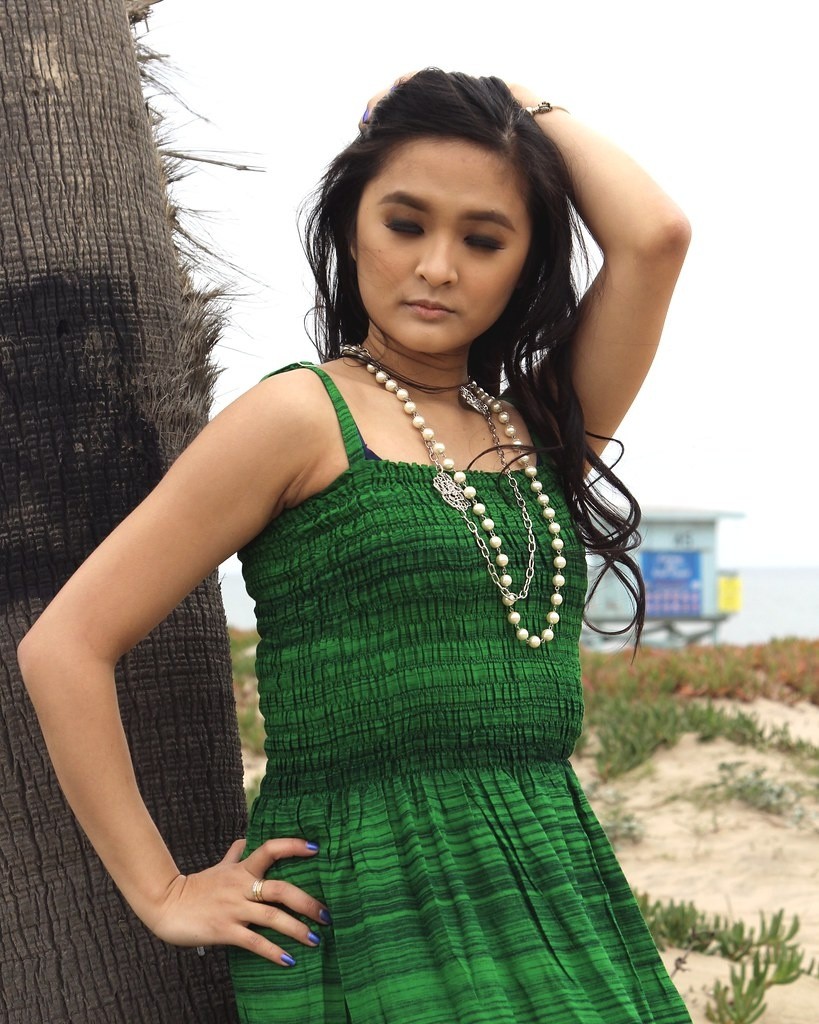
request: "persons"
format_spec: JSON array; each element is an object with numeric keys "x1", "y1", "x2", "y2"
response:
[{"x1": 17, "y1": 66, "x2": 694, "y2": 1024}]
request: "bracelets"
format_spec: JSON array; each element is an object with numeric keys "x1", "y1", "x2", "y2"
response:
[{"x1": 525, "y1": 101, "x2": 569, "y2": 117}]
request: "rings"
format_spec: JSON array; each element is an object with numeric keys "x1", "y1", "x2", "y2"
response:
[{"x1": 253, "y1": 879, "x2": 265, "y2": 902}]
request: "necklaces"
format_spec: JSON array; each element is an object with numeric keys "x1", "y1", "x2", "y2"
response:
[{"x1": 341, "y1": 343, "x2": 566, "y2": 648}]
